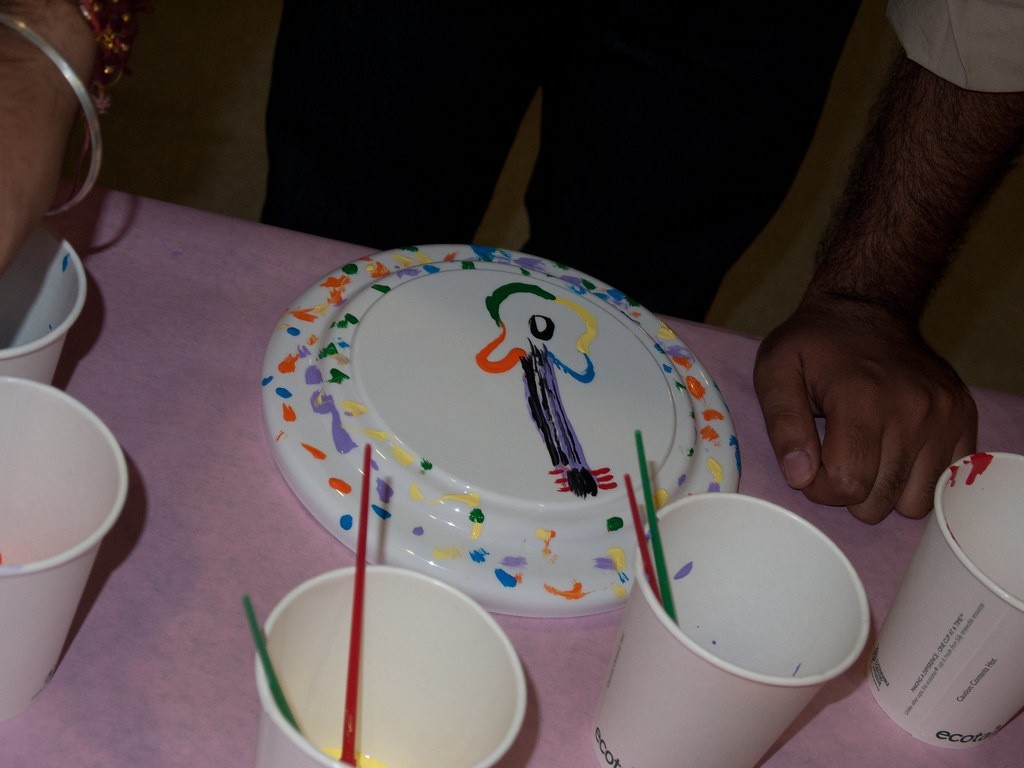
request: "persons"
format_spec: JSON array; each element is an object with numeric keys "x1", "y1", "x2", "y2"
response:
[{"x1": 0, "y1": 2, "x2": 1022, "y2": 526}]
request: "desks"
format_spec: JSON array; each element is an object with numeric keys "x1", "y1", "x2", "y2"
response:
[{"x1": 1, "y1": 168, "x2": 1024, "y2": 767}]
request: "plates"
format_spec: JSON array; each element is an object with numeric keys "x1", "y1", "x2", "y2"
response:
[{"x1": 262, "y1": 244, "x2": 738, "y2": 617}]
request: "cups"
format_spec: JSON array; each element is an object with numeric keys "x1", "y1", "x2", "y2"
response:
[
  {"x1": 253, "y1": 565, "x2": 526, "y2": 768},
  {"x1": 0, "y1": 376, "x2": 127, "y2": 722},
  {"x1": 0, "y1": 224, "x2": 87, "y2": 387},
  {"x1": 592, "y1": 494, "x2": 870, "y2": 768},
  {"x1": 870, "y1": 453, "x2": 1024, "y2": 747}
]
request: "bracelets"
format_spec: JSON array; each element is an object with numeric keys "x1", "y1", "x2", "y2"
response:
[
  {"x1": 1, "y1": 13, "x2": 103, "y2": 218},
  {"x1": 67, "y1": 2, "x2": 132, "y2": 161}
]
